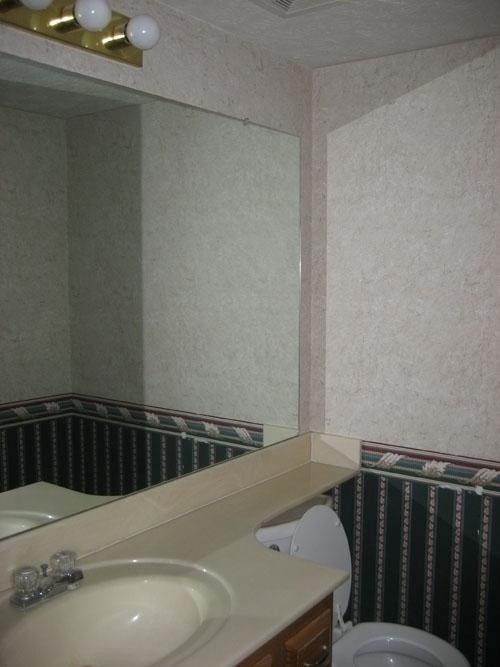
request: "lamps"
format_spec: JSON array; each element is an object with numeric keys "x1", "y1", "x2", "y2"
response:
[{"x1": 0, "y1": 0, "x2": 162, "y2": 69}]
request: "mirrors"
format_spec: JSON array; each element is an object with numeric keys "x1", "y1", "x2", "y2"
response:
[{"x1": 0, "y1": 47, "x2": 304, "y2": 543}]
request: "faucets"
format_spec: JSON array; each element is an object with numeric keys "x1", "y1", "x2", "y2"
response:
[{"x1": 8, "y1": 549, "x2": 86, "y2": 614}]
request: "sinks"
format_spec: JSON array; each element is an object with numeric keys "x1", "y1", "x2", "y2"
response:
[
  {"x1": 0, "y1": 557, "x2": 233, "y2": 667},
  {"x1": 0, "y1": 510, "x2": 56, "y2": 539}
]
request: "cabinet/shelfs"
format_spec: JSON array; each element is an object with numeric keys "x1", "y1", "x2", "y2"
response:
[{"x1": 234, "y1": 590, "x2": 334, "y2": 667}]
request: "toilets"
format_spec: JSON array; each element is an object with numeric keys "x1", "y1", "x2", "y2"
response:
[{"x1": 255, "y1": 494, "x2": 471, "y2": 667}]
